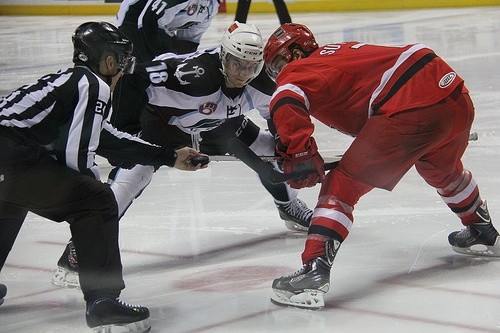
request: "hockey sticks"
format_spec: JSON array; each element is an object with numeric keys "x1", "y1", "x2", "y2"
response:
[
  {"x1": 259, "y1": 132, "x2": 478, "y2": 184},
  {"x1": 206, "y1": 155, "x2": 347, "y2": 171}
]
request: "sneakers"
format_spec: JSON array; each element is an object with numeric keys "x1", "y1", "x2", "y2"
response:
[
  {"x1": 270, "y1": 239, "x2": 342, "y2": 311},
  {"x1": 0, "y1": 284, "x2": 7, "y2": 305},
  {"x1": 85, "y1": 296, "x2": 152, "y2": 333},
  {"x1": 273, "y1": 189, "x2": 316, "y2": 233},
  {"x1": 448, "y1": 199, "x2": 500, "y2": 258},
  {"x1": 50, "y1": 238, "x2": 81, "y2": 290}
]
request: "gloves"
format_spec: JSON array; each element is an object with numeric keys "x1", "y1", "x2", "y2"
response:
[{"x1": 276, "y1": 137, "x2": 325, "y2": 189}]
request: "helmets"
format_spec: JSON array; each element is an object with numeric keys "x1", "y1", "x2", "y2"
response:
[
  {"x1": 71, "y1": 21, "x2": 136, "y2": 74},
  {"x1": 263, "y1": 23, "x2": 319, "y2": 84},
  {"x1": 219, "y1": 21, "x2": 264, "y2": 87}
]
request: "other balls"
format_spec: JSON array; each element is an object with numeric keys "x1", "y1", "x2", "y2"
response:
[{"x1": 190, "y1": 156, "x2": 210, "y2": 167}]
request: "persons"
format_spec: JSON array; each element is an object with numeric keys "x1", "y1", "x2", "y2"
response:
[
  {"x1": 51, "y1": 21, "x2": 314, "y2": 290},
  {"x1": 262, "y1": 23, "x2": 500, "y2": 310},
  {"x1": 0, "y1": 22, "x2": 208, "y2": 333},
  {"x1": 107, "y1": 0, "x2": 219, "y2": 134}
]
